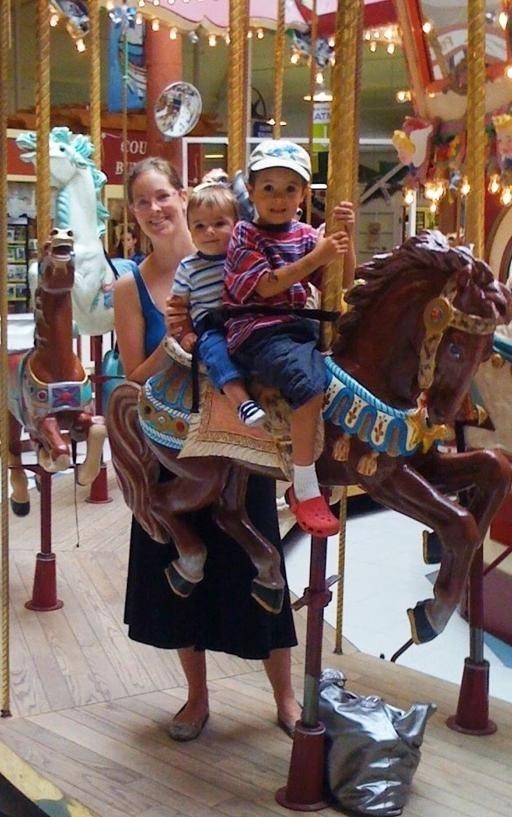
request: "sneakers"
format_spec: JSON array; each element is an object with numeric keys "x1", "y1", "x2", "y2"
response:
[{"x1": 236, "y1": 399, "x2": 267, "y2": 428}]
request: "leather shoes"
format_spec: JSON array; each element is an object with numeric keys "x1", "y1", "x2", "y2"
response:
[
  {"x1": 277, "y1": 698, "x2": 304, "y2": 738},
  {"x1": 169, "y1": 700, "x2": 209, "y2": 742}
]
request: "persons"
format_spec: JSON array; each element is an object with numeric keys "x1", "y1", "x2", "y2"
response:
[
  {"x1": 221, "y1": 140, "x2": 356, "y2": 539},
  {"x1": 114, "y1": 227, "x2": 144, "y2": 266},
  {"x1": 171, "y1": 182, "x2": 266, "y2": 427},
  {"x1": 112, "y1": 158, "x2": 306, "y2": 742}
]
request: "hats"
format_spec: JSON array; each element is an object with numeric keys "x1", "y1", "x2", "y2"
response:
[{"x1": 247, "y1": 138, "x2": 313, "y2": 183}]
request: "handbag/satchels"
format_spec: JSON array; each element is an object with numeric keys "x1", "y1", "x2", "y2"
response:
[{"x1": 317, "y1": 668, "x2": 437, "y2": 817}]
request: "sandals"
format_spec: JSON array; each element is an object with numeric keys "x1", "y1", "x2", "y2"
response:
[{"x1": 285, "y1": 483, "x2": 341, "y2": 537}]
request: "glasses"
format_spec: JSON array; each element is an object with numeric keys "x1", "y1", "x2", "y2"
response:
[{"x1": 128, "y1": 185, "x2": 182, "y2": 214}]
